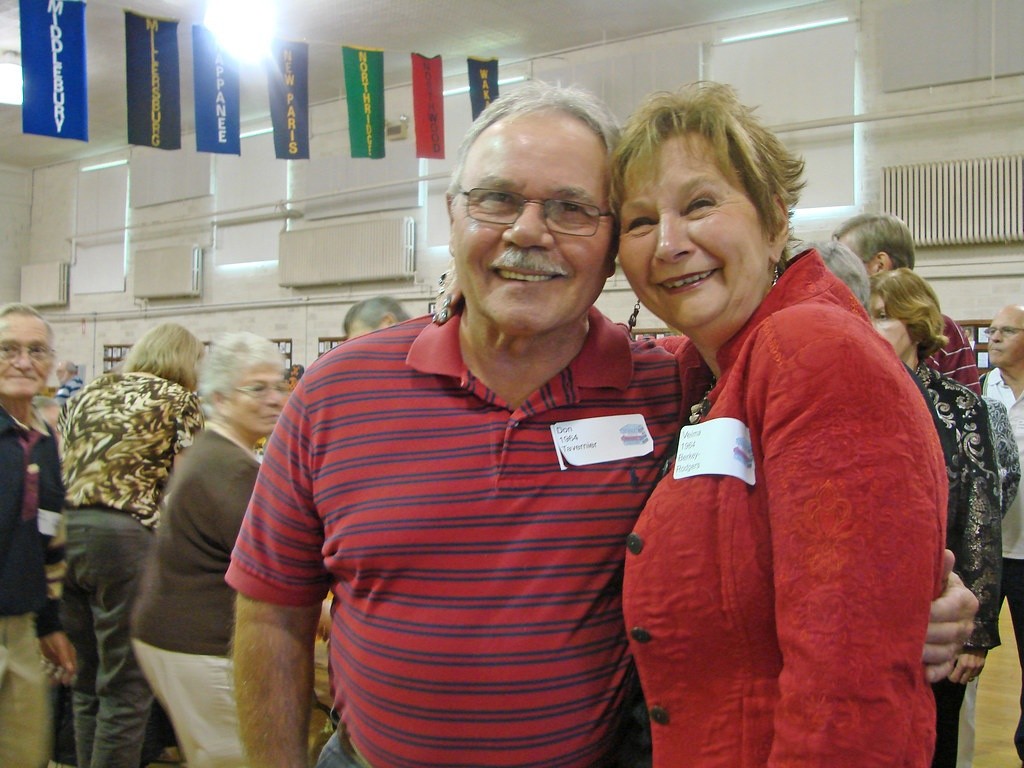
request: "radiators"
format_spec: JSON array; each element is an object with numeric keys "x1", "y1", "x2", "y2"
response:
[
  {"x1": 133, "y1": 244, "x2": 202, "y2": 299},
  {"x1": 879, "y1": 154, "x2": 1024, "y2": 247},
  {"x1": 278, "y1": 216, "x2": 414, "y2": 286},
  {"x1": 20, "y1": 261, "x2": 67, "y2": 307}
]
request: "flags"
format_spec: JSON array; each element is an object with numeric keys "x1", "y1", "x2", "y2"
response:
[{"x1": 19, "y1": 0, "x2": 498, "y2": 159}]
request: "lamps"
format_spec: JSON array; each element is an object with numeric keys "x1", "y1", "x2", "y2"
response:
[{"x1": 385, "y1": 114, "x2": 408, "y2": 141}]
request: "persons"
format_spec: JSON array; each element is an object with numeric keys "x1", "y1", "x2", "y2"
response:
[
  {"x1": 59, "y1": 323, "x2": 205, "y2": 768},
  {"x1": 799, "y1": 212, "x2": 1022, "y2": 767},
  {"x1": 54, "y1": 361, "x2": 82, "y2": 401},
  {"x1": 129, "y1": 335, "x2": 287, "y2": 768},
  {"x1": 345, "y1": 297, "x2": 406, "y2": 341},
  {"x1": 223, "y1": 85, "x2": 979, "y2": 768},
  {"x1": 0, "y1": 303, "x2": 78, "y2": 768},
  {"x1": 609, "y1": 84, "x2": 949, "y2": 767},
  {"x1": 980, "y1": 305, "x2": 1024, "y2": 768}
]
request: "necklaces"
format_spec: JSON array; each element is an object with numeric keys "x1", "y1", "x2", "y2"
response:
[{"x1": 689, "y1": 378, "x2": 715, "y2": 423}]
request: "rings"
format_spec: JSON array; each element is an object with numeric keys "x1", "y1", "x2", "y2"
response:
[{"x1": 969, "y1": 676, "x2": 975, "y2": 681}]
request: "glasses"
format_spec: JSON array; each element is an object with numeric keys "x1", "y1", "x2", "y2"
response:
[
  {"x1": 460, "y1": 188, "x2": 611, "y2": 237},
  {"x1": 984, "y1": 327, "x2": 1024, "y2": 336},
  {"x1": 230, "y1": 385, "x2": 287, "y2": 400}
]
